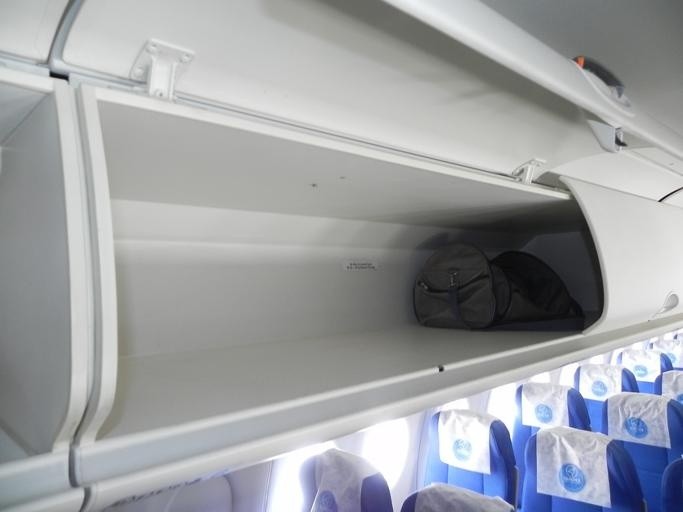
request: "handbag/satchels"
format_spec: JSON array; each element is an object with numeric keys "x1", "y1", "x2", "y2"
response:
[{"x1": 413, "y1": 240, "x2": 584, "y2": 331}]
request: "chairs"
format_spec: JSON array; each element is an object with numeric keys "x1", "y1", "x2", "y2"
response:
[
  {"x1": 601, "y1": 392, "x2": 683, "y2": 450},
  {"x1": 402, "y1": 484, "x2": 512, "y2": 511},
  {"x1": 575, "y1": 338, "x2": 683, "y2": 429},
  {"x1": 521, "y1": 428, "x2": 644, "y2": 508},
  {"x1": 301, "y1": 450, "x2": 393, "y2": 510},
  {"x1": 421, "y1": 410, "x2": 516, "y2": 500},
  {"x1": 516, "y1": 382, "x2": 593, "y2": 469},
  {"x1": 661, "y1": 455, "x2": 682, "y2": 510}
]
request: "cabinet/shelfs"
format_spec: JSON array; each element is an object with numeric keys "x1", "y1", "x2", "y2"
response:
[
  {"x1": 0, "y1": 70, "x2": 93, "y2": 512},
  {"x1": 75, "y1": 78, "x2": 683, "y2": 512}
]
